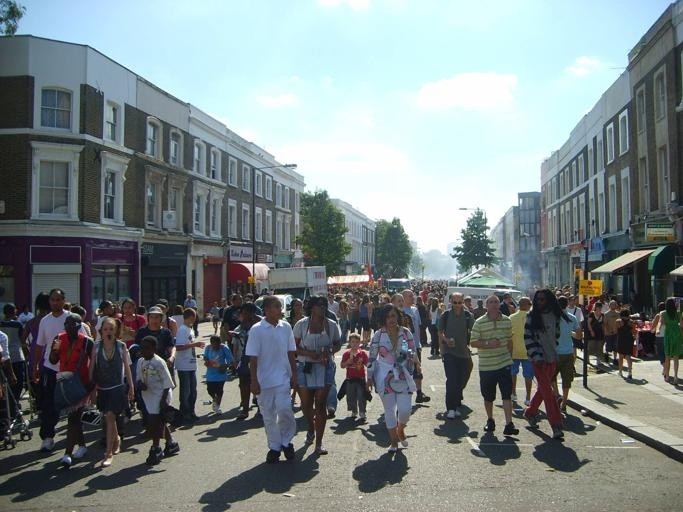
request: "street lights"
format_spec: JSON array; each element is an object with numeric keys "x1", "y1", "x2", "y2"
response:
[
  {"x1": 458, "y1": 206, "x2": 489, "y2": 269},
  {"x1": 252, "y1": 164, "x2": 299, "y2": 302}
]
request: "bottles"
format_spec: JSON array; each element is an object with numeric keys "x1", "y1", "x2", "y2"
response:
[
  {"x1": 449, "y1": 337, "x2": 454, "y2": 345},
  {"x1": 402, "y1": 340, "x2": 408, "y2": 352}
]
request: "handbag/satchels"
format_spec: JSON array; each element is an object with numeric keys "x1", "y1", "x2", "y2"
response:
[
  {"x1": 302, "y1": 361, "x2": 326, "y2": 391},
  {"x1": 80, "y1": 400, "x2": 103, "y2": 427},
  {"x1": 54, "y1": 369, "x2": 86, "y2": 410}
]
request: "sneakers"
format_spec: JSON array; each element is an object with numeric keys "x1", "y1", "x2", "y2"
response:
[
  {"x1": 415, "y1": 394, "x2": 430, "y2": 403},
  {"x1": 212, "y1": 400, "x2": 221, "y2": 416},
  {"x1": 396, "y1": 427, "x2": 409, "y2": 447},
  {"x1": 267, "y1": 450, "x2": 280, "y2": 463},
  {"x1": 446, "y1": 411, "x2": 565, "y2": 438},
  {"x1": 512, "y1": 395, "x2": 534, "y2": 405},
  {"x1": 282, "y1": 444, "x2": 296, "y2": 461},
  {"x1": 41, "y1": 437, "x2": 179, "y2": 466},
  {"x1": 388, "y1": 443, "x2": 398, "y2": 452}
]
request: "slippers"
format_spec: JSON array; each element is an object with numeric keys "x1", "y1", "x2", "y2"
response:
[
  {"x1": 316, "y1": 446, "x2": 329, "y2": 455},
  {"x1": 305, "y1": 431, "x2": 315, "y2": 443}
]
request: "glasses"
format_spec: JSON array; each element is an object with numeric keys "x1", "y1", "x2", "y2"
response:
[
  {"x1": 535, "y1": 297, "x2": 548, "y2": 302},
  {"x1": 312, "y1": 302, "x2": 329, "y2": 306},
  {"x1": 453, "y1": 300, "x2": 463, "y2": 305},
  {"x1": 65, "y1": 322, "x2": 81, "y2": 328}
]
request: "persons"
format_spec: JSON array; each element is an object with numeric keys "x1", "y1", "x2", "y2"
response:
[
  {"x1": 246, "y1": 297, "x2": 295, "y2": 461},
  {"x1": 650, "y1": 302, "x2": 667, "y2": 377},
  {"x1": 523, "y1": 288, "x2": 566, "y2": 438},
  {"x1": 656, "y1": 299, "x2": 683, "y2": 385},
  {"x1": 367, "y1": 303, "x2": 418, "y2": 452},
  {"x1": 1, "y1": 288, "x2": 204, "y2": 470},
  {"x1": 203, "y1": 282, "x2": 638, "y2": 420},
  {"x1": 470, "y1": 295, "x2": 520, "y2": 436}
]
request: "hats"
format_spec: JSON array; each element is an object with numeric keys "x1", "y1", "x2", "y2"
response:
[
  {"x1": 143, "y1": 307, "x2": 167, "y2": 323},
  {"x1": 160, "y1": 406, "x2": 184, "y2": 428}
]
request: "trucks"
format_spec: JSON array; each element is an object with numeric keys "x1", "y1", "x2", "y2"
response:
[
  {"x1": 267, "y1": 266, "x2": 327, "y2": 306},
  {"x1": 445, "y1": 287, "x2": 525, "y2": 309}
]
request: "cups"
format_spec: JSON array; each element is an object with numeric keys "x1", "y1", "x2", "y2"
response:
[
  {"x1": 55, "y1": 339, "x2": 61, "y2": 350},
  {"x1": 321, "y1": 346, "x2": 327, "y2": 366}
]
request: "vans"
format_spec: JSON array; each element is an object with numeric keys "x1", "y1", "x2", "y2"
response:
[{"x1": 384, "y1": 278, "x2": 412, "y2": 294}]
